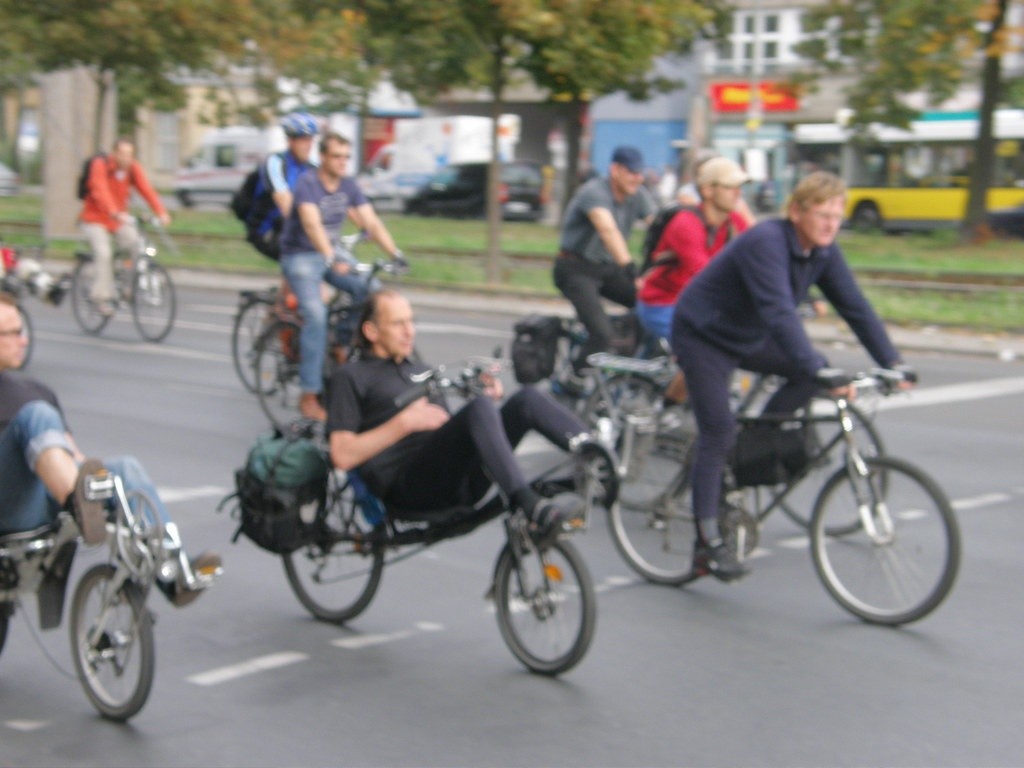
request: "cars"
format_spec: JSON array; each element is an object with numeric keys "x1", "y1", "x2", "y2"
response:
[{"x1": 405, "y1": 156, "x2": 547, "y2": 223}]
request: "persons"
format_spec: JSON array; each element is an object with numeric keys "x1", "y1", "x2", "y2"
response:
[
  {"x1": 555, "y1": 146, "x2": 657, "y2": 393},
  {"x1": 325, "y1": 288, "x2": 619, "y2": 553},
  {"x1": 680, "y1": 147, "x2": 758, "y2": 227},
  {"x1": 254, "y1": 112, "x2": 319, "y2": 260},
  {"x1": 278, "y1": 132, "x2": 409, "y2": 422},
  {"x1": 675, "y1": 172, "x2": 914, "y2": 582},
  {"x1": 0, "y1": 291, "x2": 223, "y2": 608},
  {"x1": 640, "y1": 159, "x2": 754, "y2": 407},
  {"x1": 80, "y1": 138, "x2": 172, "y2": 317}
]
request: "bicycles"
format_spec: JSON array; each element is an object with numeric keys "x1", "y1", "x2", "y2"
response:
[
  {"x1": 228, "y1": 225, "x2": 425, "y2": 438},
  {"x1": 1, "y1": 469, "x2": 229, "y2": 724},
  {"x1": 278, "y1": 342, "x2": 629, "y2": 678},
  {"x1": 493, "y1": 275, "x2": 891, "y2": 538},
  {"x1": 67, "y1": 207, "x2": 180, "y2": 343},
  {"x1": 577, "y1": 334, "x2": 963, "y2": 629}
]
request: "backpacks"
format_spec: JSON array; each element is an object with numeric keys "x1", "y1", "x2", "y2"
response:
[{"x1": 232, "y1": 172, "x2": 263, "y2": 218}]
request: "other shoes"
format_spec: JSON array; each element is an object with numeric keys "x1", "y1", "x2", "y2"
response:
[
  {"x1": 170, "y1": 552, "x2": 221, "y2": 606},
  {"x1": 530, "y1": 496, "x2": 582, "y2": 543},
  {"x1": 692, "y1": 540, "x2": 745, "y2": 580},
  {"x1": 71, "y1": 459, "x2": 107, "y2": 544}
]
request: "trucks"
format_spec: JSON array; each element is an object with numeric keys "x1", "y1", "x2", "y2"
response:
[
  {"x1": 169, "y1": 112, "x2": 358, "y2": 210},
  {"x1": 352, "y1": 113, "x2": 523, "y2": 209}
]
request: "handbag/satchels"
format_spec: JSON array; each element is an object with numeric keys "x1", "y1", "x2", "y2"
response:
[
  {"x1": 235, "y1": 436, "x2": 327, "y2": 553},
  {"x1": 509, "y1": 314, "x2": 559, "y2": 384}
]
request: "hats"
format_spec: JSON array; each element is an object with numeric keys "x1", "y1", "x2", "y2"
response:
[
  {"x1": 697, "y1": 157, "x2": 753, "y2": 188},
  {"x1": 614, "y1": 145, "x2": 646, "y2": 174}
]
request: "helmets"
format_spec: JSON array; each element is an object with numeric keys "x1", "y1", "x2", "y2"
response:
[{"x1": 282, "y1": 113, "x2": 320, "y2": 138}]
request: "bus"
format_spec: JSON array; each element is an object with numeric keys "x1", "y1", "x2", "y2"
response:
[{"x1": 763, "y1": 105, "x2": 1024, "y2": 243}]
